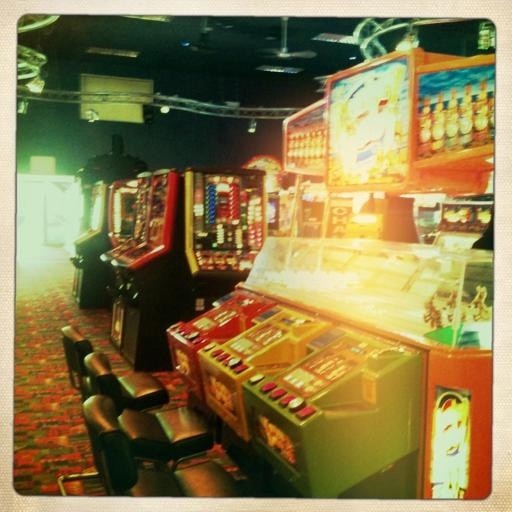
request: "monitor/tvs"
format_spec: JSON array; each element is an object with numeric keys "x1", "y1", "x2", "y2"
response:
[
  {"x1": 194, "y1": 173, "x2": 264, "y2": 250},
  {"x1": 90, "y1": 195, "x2": 101, "y2": 231},
  {"x1": 134, "y1": 183, "x2": 150, "y2": 240},
  {"x1": 147, "y1": 181, "x2": 171, "y2": 239},
  {"x1": 112, "y1": 188, "x2": 137, "y2": 235}
]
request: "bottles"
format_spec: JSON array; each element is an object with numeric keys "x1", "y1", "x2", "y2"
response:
[
  {"x1": 418, "y1": 79, "x2": 489, "y2": 142},
  {"x1": 288, "y1": 129, "x2": 327, "y2": 160}
]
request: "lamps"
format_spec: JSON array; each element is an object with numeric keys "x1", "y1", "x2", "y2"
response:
[
  {"x1": 247, "y1": 115, "x2": 257, "y2": 133},
  {"x1": 17, "y1": 98, "x2": 30, "y2": 115},
  {"x1": 160, "y1": 105, "x2": 171, "y2": 114}
]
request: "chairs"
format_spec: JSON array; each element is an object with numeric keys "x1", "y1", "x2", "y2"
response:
[{"x1": 56, "y1": 324, "x2": 250, "y2": 498}]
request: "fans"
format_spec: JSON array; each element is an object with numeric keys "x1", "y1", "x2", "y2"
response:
[{"x1": 257, "y1": 17, "x2": 316, "y2": 60}]
